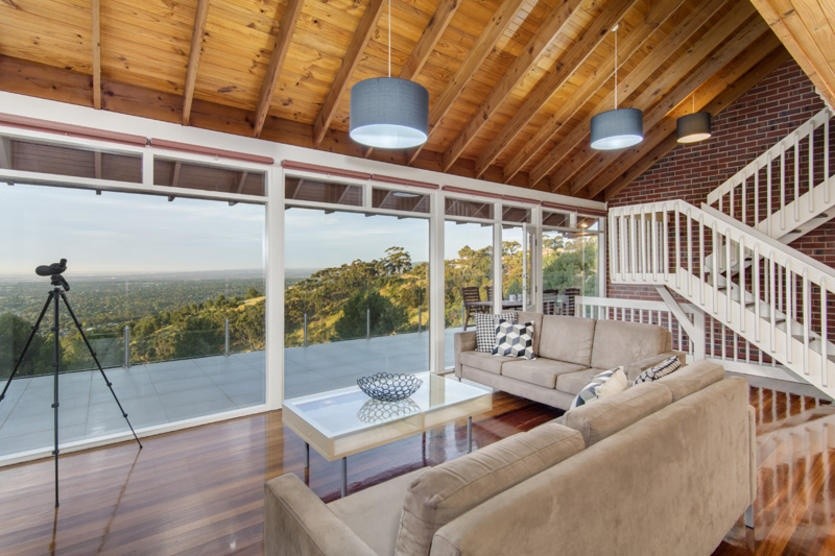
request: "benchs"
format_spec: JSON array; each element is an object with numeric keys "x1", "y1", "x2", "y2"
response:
[
  {"x1": 265, "y1": 360, "x2": 757, "y2": 556},
  {"x1": 454, "y1": 310, "x2": 685, "y2": 413}
]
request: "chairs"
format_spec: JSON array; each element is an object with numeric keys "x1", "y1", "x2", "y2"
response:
[
  {"x1": 462, "y1": 287, "x2": 484, "y2": 331},
  {"x1": 563, "y1": 288, "x2": 579, "y2": 315},
  {"x1": 484, "y1": 285, "x2": 504, "y2": 301},
  {"x1": 542, "y1": 288, "x2": 559, "y2": 315}
]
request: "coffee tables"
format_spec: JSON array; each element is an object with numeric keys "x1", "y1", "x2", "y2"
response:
[{"x1": 282, "y1": 372, "x2": 492, "y2": 502}]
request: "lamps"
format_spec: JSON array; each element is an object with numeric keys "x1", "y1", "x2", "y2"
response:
[
  {"x1": 677, "y1": 95, "x2": 711, "y2": 143},
  {"x1": 349, "y1": 0, "x2": 429, "y2": 150},
  {"x1": 590, "y1": 23, "x2": 643, "y2": 151}
]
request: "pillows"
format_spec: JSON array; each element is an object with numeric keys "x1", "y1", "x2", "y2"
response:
[
  {"x1": 570, "y1": 365, "x2": 627, "y2": 409},
  {"x1": 491, "y1": 318, "x2": 536, "y2": 361},
  {"x1": 633, "y1": 355, "x2": 681, "y2": 386},
  {"x1": 474, "y1": 312, "x2": 518, "y2": 352}
]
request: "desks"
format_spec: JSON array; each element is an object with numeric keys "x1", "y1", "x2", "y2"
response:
[{"x1": 465, "y1": 300, "x2": 522, "y2": 313}]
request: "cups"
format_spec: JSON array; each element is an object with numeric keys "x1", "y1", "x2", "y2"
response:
[
  {"x1": 518, "y1": 294, "x2": 522, "y2": 302},
  {"x1": 509, "y1": 294, "x2": 516, "y2": 301}
]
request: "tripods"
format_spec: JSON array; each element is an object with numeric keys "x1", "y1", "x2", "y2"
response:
[{"x1": 0, "y1": 289, "x2": 142, "y2": 508}]
